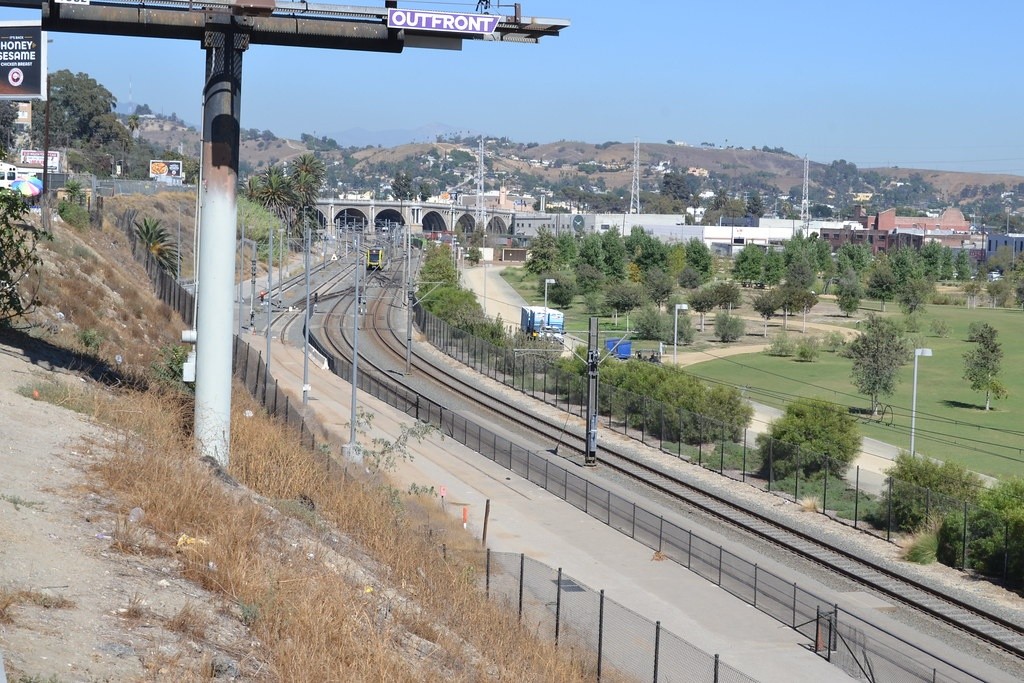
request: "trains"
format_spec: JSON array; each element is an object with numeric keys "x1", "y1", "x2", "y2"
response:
[{"x1": 366, "y1": 244, "x2": 382, "y2": 270}]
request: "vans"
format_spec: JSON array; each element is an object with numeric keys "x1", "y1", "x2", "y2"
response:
[{"x1": 0, "y1": 162, "x2": 17, "y2": 190}]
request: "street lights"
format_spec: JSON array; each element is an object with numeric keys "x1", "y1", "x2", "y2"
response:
[
  {"x1": 406, "y1": 192, "x2": 410, "y2": 201},
  {"x1": 909, "y1": 347, "x2": 934, "y2": 459},
  {"x1": 482, "y1": 234, "x2": 487, "y2": 265},
  {"x1": 674, "y1": 304, "x2": 688, "y2": 366},
  {"x1": 543, "y1": 278, "x2": 556, "y2": 331},
  {"x1": 239, "y1": 213, "x2": 250, "y2": 303},
  {"x1": 302, "y1": 206, "x2": 309, "y2": 263},
  {"x1": 285, "y1": 206, "x2": 294, "y2": 250}
]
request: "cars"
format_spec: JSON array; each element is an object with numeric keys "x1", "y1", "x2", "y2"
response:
[{"x1": 987, "y1": 272, "x2": 1000, "y2": 278}]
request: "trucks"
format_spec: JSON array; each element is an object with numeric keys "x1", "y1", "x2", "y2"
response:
[{"x1": 520, "y1": 306, "x2": 566, "y2": 347}]
